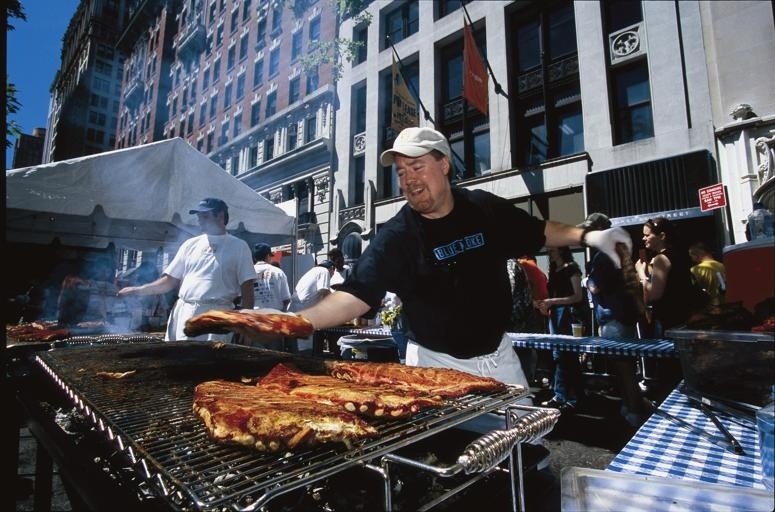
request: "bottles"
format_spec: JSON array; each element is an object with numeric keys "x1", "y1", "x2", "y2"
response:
[
  {"x1": 757, "y1": 384, "x2": 775, "y2": 492},
  {"x1": 747, "y1": 202, "x2": 774, "y2": 241}
]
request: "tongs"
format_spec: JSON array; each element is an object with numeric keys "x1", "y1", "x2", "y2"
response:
[
  {"x1": 684, "y1": 387, "x2": 775, "y2": 435},
  {"x1": 650, "y1": 396, "x2": 744, "y2": 455}
]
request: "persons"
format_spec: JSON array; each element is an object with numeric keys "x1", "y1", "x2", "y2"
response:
[
  {"x1": 287, "y1": 259, "x2": 335, "y2": 354},
  {"x1": 116, "y1": 198, "x2": 258, "y2": 344},
  {"x1": 250, "y1": 242, "x2": 290, "y2": 353},
  {"x1": 244, "y1": 125, "x2": 631, "y2": 472},
  {"x1": 328, "y1": 246, "x2": 351, "y2": 292}
]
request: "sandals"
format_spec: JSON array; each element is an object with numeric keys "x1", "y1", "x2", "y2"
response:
[{"x1": 541, "y1": 395, "x2": 581, "y2": 416}]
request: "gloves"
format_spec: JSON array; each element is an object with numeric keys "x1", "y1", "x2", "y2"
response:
[
  {"x1": 239, "y1": 307, "x2": 297, "y2": 318},
  {"x1": 584, "y1": 226, "x2": 633, "y2": 270}
]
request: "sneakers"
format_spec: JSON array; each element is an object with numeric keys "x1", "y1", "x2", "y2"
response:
[{"x1": 621, "y1": 397, "x2": 653, "y2": 426}]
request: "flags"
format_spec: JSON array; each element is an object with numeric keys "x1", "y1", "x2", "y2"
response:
[
  {"x1": 391, "y1": 53, "x2": 419, "y2": 133},
  {"x1": 462, "y1": 16, "x2": 489, "y2": 116}
]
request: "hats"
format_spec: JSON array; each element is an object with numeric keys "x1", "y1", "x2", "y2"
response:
[
  {"x1": 379, "y1": 127, "x2": 451, "y2": 168},
  {"x1": 318, "y1": 259, "x2": 335, "y2": 270},
  {"x1": 575, "y1": 212, "x2": 612, "y2": 232},
  {"x1": 189, "y1": 198, "x2": 228, "y2": 215},
  {"x1": 253, "y1": 242, "x2": 274, "y2": 257}
]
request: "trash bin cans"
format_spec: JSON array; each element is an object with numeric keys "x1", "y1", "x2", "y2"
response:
[{"x1": 340, "y1": 335, "x2": 399, "y2": 361}]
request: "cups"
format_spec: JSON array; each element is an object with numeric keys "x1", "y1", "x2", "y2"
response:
[
  {"x1": 352, "y1": 313, "x2": 378, "y2": 326},
  {"x1": 570, "y1": 322, "x2": 586, "y2": 337}
]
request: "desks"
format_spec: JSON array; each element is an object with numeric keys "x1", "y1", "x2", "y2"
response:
[
  {"x1": 321, "y1": 319, "x2": 674, "y2": 444},
  {"x1": 566, "y1": 369, "x2": 774, "y2": 511}
]
